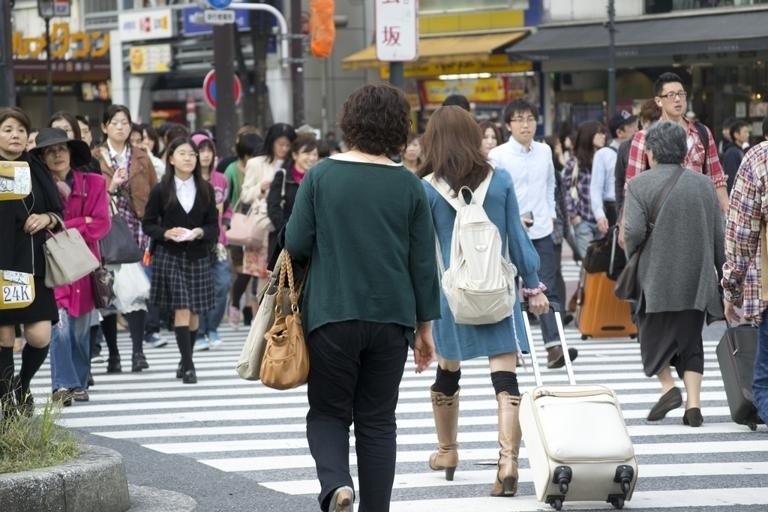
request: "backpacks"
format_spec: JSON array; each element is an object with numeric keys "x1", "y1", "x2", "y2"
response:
[{"x1": 420, "y1": 163, "x2": 517, "y2": 324}]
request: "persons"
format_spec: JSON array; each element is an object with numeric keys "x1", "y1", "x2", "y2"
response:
[
  {"x1": 277, "y1": 82, "x2": 444, "y2": 512},
  {"x1": 409, "y1": 105, "x2": 553, "y2": 496}
]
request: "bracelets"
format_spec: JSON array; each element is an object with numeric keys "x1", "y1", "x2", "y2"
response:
[{"x1": 520, "y1": 282, "x2": 546, "y2": 296}]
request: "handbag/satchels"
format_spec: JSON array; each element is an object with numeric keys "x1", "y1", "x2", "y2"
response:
[
  {"x1": 259, "y1": 317, "x2": 310, "y2": 390},
  {"x1": 614, "y1": 253, "x2": 639, "y2": 299},
  {"x1": 0, "y1": 269, "x2": 36, "y2": 310},
  {"x1": 585, "y1": 236, "x2": 613, "y2": 274},
  {"x1": 0, "y1": 160, "x2": 33, "y2": 202},
  {"x1": 236, "y1": 286, "x2": 294, "y2": 382},
  {"x1": 606, "y1": 224, "x2": 626, "y2": 277},
  {"x1": 91, "y1": 267, "x2": 119, "y2": 310},
  {"x1": 224, "y1": 211, "x2": 263, "y2": 247},
  {"x1": 568, "y1": 185, "x2": 579, "y2": 207},
  {"x1": 99, "y1": 215, "x2": 143, "y2": 265},
  {"x1": 43, "y1": 226, "x2": 100, "y2": 290}
]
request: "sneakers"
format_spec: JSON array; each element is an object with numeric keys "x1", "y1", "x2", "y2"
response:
[
  {"x1": 646, "y1": 387, "x2": 682, "y2": 422},
  {"x1": 681, "y1": 410, "x2": 703, "y2": 426},
  {"x1": 528, "y1": 314, "x2": 577, "y2": 369},
  {"x1": 229, "y1": 303, "x2": 253, "y2": 327},
  {"x1": 0, "y1": 330, "x2": 224, "y2": 424}
]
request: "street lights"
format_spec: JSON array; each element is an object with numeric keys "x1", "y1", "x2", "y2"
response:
[{"x1": 35, "y1": 0, "x2": 56, "y2": 117}]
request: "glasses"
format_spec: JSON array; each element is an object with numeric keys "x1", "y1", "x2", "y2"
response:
[
  {"x1": 657, "y1": 89, "x2": 689, "y2": 99},
  {"x1": 507, "y1": 114, "x2": 537, "y2": 124}
]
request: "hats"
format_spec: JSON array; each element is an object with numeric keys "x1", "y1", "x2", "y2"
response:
[
  {"x1": 29, "y1": 127, "x2": 92, "y2": 168},
  {"x1": 609, "y1": 109, "x2": 637, "y2": 133}
]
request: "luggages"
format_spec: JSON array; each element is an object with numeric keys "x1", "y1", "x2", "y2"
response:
[
  {"x1": 520, "y1": 383, "x2": 638, "y2": 505},
  {"x1": 715, "y1": 322, "x2": 768, "y2": 425},
  {"x1": 575, "y1": 265, "x2": 639, "y2": 338}
]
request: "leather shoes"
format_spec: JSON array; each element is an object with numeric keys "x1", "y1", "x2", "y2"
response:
[{"x1": 327, "y1": 486, "x2": 356, "y2": 512}]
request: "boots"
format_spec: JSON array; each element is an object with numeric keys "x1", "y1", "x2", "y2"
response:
[
  {"x1": 426, "y1": 383, "x2": 464, "y2": 481},
  {"x1": 488, "y1": 389, "x2": 526, "y2": 498}
]
